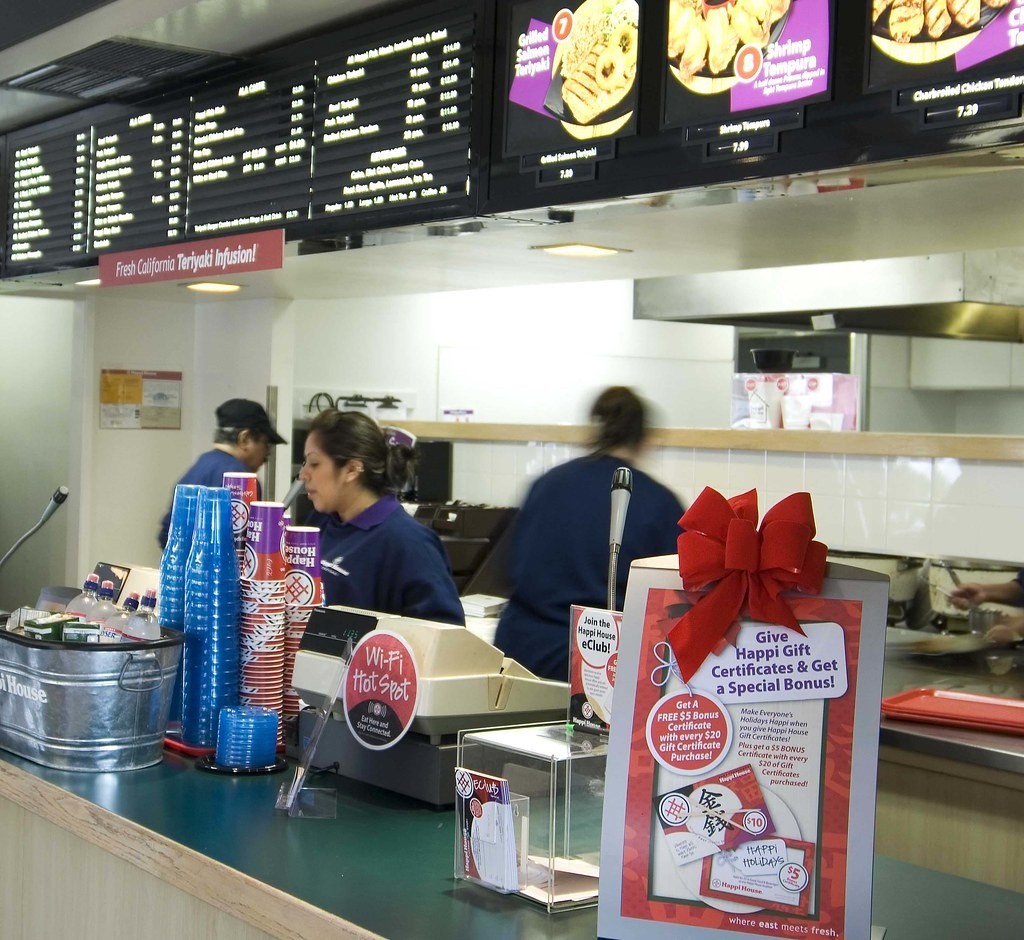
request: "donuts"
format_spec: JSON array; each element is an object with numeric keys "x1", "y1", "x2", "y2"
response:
[
  {"x1": 595, "y1": 51, "x2": 624, "y2": 88},
  {"x1": 612, "y1": 23, "x2": 638, "y2": 66}
]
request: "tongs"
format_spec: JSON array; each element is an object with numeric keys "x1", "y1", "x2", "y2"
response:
[{"x1": 920, "y1": 560, "x2": 979, "y2": 608}]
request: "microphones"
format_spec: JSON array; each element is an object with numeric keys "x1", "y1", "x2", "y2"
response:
[
  {"x1": 0, "y1": 487, "x2": 69, "y2": 566},
  {"x1": 607, "y1": 467, "x2": 632, "y2": 609}
]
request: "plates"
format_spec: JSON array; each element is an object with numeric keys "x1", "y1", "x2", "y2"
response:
[
  {"x1": 670, "y1": 12, "x2": 790, "y2": 95},
  {"x1": 873, "y1": 30, "x2": 982, "y2": 64},
  {"x1": 196, "y1": 754, "x2": 289, "y2": 775},
  {"x1": 553, "y1": 0, "x2": 639, "y2": 79}
]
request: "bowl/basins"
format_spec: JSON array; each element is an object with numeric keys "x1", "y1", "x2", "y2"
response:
[{"x1": 810, "y1": 413, "x2": 844, "y2": 430}]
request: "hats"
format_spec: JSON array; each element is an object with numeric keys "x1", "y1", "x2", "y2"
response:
[{"x1": 215, "y1": 399, "x2": 286, "y2": 444}]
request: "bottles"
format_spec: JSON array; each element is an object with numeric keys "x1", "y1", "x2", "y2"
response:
[
  {"x1": 65, "y1": 574, "x2": 99, "y2": 617},
  {"x1": 86, "y1": 580, "x2": 118, "y2": 623},
  {"x1": 119, "y1": 589, "x2": 161, "y2": 642},
  {"x1": 100, "y1": 593, "x2": 139, "y2": 643}
]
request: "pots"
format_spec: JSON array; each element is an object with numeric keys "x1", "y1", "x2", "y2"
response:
[{"x1": 828, "y1": 549, "x2": 1024, "y2": 632}]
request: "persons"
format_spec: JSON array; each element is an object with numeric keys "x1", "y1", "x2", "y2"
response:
[
  {"x1": 297, "y1": 408, "x2": 468, "y2": 629},
  {"x1": 945, "y1": 566, "x2": 1024, "y2": 643},
  {"x1": 158, "y1": 399, "x2": 290, "y2": 556},
  {"x1": 490, "y1": 386, "x2": 688, "y2": 686}
]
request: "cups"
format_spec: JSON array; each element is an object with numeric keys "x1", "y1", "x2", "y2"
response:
[
  {"x1": 748, "y1": 383, "x2": 784, "y2": 429},
  {"x1": 970, "y1": 609, "x2": 1003, "y2": 635},
  {"x1": 160, "y1": 473, "x2": 326, "y2": 748},
  {"x1": 780, "y1": 395, "x2": 813, "y2": 429}
]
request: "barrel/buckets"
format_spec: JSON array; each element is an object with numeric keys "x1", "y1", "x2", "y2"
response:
[{"x1": 0, "y1": 615, "x2": 184, "y2": 772}]
